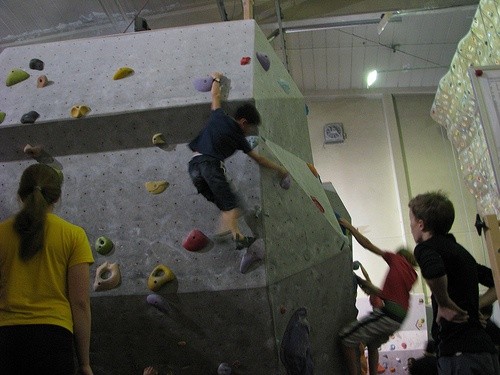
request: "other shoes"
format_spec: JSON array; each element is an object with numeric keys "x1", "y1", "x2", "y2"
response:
[
  {"x1": 234, "y1": 235, "x2": 257, "y2": 251},
  {"x1": 215, "y1": 228, "x2": 236, "y2": 239}
]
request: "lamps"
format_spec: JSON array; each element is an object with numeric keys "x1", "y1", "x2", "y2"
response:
[{"x1": 376, "y1": 12, "x2": 393, "y2": 36}]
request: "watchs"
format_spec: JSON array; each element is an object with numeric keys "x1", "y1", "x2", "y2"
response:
[{"x1": 212, "y1": 77, "x2": 221, "y2": 83}]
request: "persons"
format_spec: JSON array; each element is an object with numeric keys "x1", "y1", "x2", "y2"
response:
[
  {"x1": 143, "y1": 366, "x2": 158, "y2": 375},
  {"x1": 0, "y1": 164, "x2": 95, "y2": 375},
  {"x1": 339, "y1": 192, "x2": 500, "y2": 375},
  {"x1": 182, "y1": 71, "x2": 288, "y2": 250}
]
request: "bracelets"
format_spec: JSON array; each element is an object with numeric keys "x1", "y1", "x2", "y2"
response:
[{"x1": 352, "y1": 230, "x2": 359, "y2": 235}]
